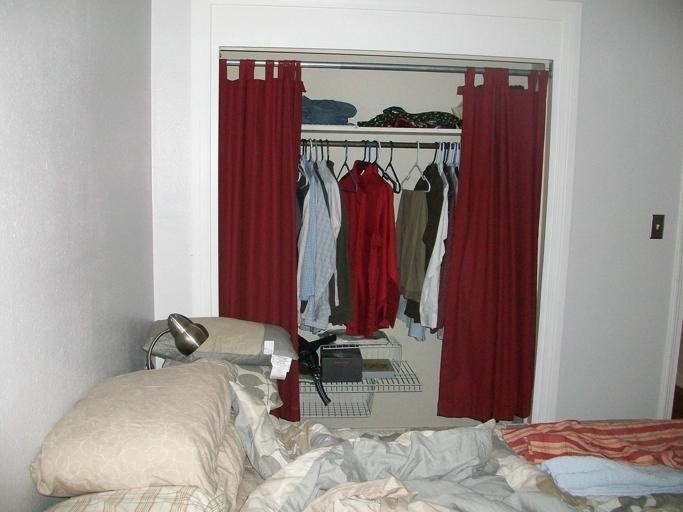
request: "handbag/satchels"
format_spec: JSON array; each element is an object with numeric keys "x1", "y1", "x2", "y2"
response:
[{"x1": 299, "y1": 334, "x2": 319, "y2": 374}]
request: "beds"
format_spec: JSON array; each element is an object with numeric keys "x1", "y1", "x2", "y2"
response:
[{"x1": 241, "y1": 417, "x2": 682, "y2": 511}]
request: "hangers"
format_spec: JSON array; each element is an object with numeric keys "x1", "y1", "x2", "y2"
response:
[{"x1": 296, "y1": 138, "x2": 460, "y2": 194}]
